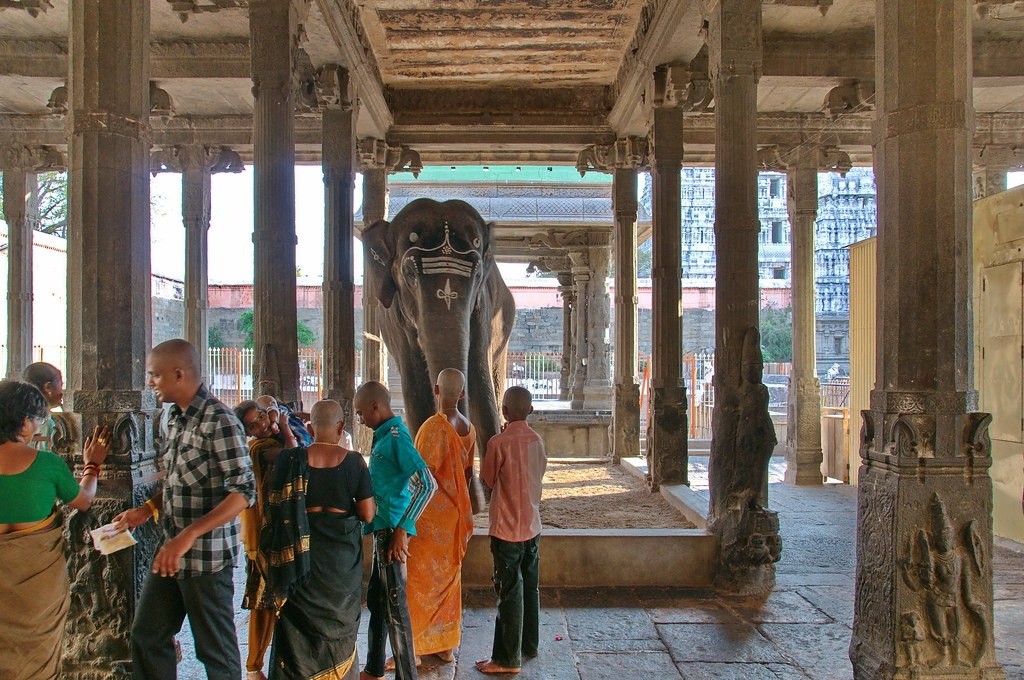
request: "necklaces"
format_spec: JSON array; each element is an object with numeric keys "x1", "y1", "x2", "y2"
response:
[
  {"x1": 312, "y1": 442, "x2": 338, "y2": 446},
  {"x1": 444, "y1": 408, "x2": 457, "y2": 411}
]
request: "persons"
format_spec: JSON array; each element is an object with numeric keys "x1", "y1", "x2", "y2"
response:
[
  {"x1": 385, "y1": 369, "x2": 476, "y2": 671},
  {"x1": 0, "y1": 361, "x2": 111, "y2": 680},
  {"x1": 112, "y1": 338, "x2": 256, "y2": 680},
  {"x1": 234, "y1": 395, "x2": 354, "y2": 680},
  {"x1": 474, "y1": 386, "x2": 548, "y2": 674},
  {"x1": 263, "y1": 399, "x2": 376, "y2": 680},
  {"x1": 354, "y1": 381, "x2": 439, "y2": 680}
]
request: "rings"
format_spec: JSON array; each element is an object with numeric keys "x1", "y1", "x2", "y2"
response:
[
  {"x1": 98, "y1": 438, "x2": 103, "y2": 443},
  {"x1": 101, "y1": 443, "x2": 106, "y2": 445}
]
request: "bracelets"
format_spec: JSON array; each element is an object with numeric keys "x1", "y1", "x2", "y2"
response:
[
  {"x1": 82, "y1": 473, "x2": 97, "y2": 478},
  {"x1": 145, "y1": 500, "x2": 158, "y2": 524},
  {"x1": 83, "y1": 462, "x2": 100, "y2": 473}
]
